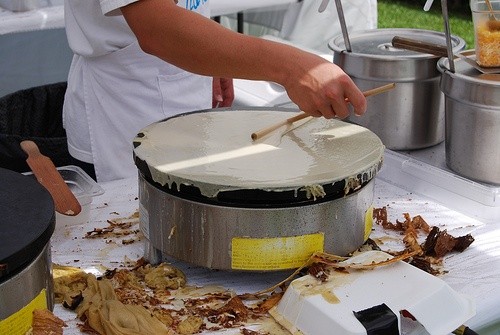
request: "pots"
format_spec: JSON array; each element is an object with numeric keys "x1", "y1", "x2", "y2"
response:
[
  {"x1": 436, "y1": 49, "x2": 500, "y2": 186},
  {"x1": 327, "y1": 27, "x2": 466, "y2": 151}
]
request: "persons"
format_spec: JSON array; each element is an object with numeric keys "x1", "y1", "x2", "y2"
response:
[{"x1": 63, "y1": 1, "x2": 368, "y2": 182}]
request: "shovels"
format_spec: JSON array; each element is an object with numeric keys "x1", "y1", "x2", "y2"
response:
[{"x1": 392, "y1": 35, "x2": 500, "y2": 74}]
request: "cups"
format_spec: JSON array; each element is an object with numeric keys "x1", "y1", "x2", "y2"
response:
[{"x1": 468, "y1": 0, "x2": 500, "y2": 69}]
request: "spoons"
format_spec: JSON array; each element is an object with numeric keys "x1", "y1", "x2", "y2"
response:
[{"x1": 485, "y1": 0, "x2": 500, "y2": 31}]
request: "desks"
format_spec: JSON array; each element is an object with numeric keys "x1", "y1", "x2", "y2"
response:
[
  {"x1": 0, "y1": 0, "x2": 291, "y2": 35},
  {"x1": 51, "y1": 144, "x2": 500, "y2": 335}
]
